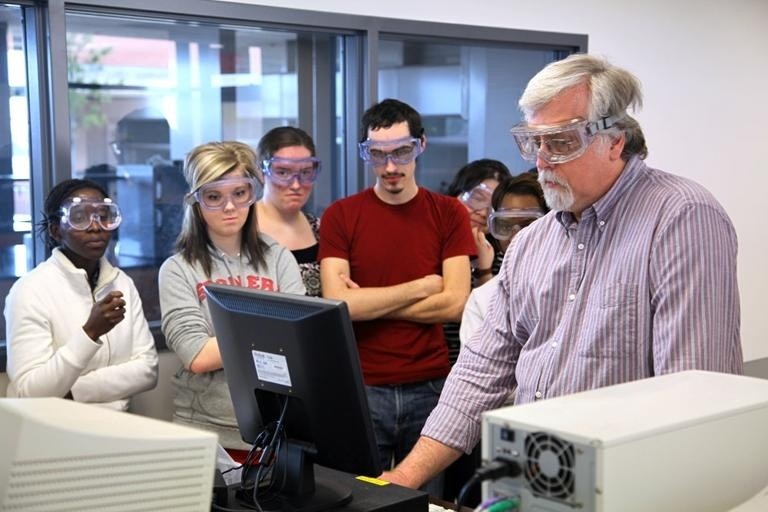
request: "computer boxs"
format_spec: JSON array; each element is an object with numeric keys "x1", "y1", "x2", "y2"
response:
[
  {"x1": 480, "y1": 368, "x2": 768, "y2": 512},
  {"x1": 215, "y1": 459, "x2": 430, "y2": 512}
]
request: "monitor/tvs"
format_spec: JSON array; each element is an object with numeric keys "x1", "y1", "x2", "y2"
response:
[{"x1": 202, "y1": 283, "x2": 384, "y2": 512}]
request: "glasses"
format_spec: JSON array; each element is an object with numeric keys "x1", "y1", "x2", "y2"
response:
[
  {"x1": 488, "y1": 207, "x2": 545, "y2": 241},
  {"x1": 358, "y1": 137, "x2": 422, "y2": 166},
  {"x1": 461, "y1": 184, "x2": 494, "y2": 215},
  {"x1": 194, "y1": 177, "x2": 261, "y2": 211},
  {"x1": 58, "y1": 198, "x2": 122, "y2": 233},
  {"x1": 263, "y1": 158, "x2": 321, "y2": 185},
  {"x1": 510, "y1": 115, "x2": 613, "y2": 165}
]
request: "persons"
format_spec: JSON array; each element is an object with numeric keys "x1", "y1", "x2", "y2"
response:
[
  {"x1": 110, "y1": 107, "x2": 189, "y2": 263},
  {"x1": 470, "y1": 172, "x2": 549, "y2": 287},
  {"x1": 254, "y1": 126, "x2": 322, "y2": 300},
  {"x1": 157, "y1": 141, "x2": 308, "y2": 466},
  {"x1": 85, "y1": 164, "x2": 118, "y2": 195},
  {"x1": 4, "y1": 179, "x2": 158, "y2": 413},
  {"x1": 315, "y1": 99, "x2": 479, "y2": 499},
  {"x1": 378, "y1": 55, "x2": 743, "y2": 490},
  {"x1": 441, "y1": 160, "x2": 513, "y2": 366}
]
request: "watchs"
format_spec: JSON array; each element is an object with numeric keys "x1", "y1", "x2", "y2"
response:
[{"x1": 470, "y1": 267, "x2": 492, "y2": 278}]
